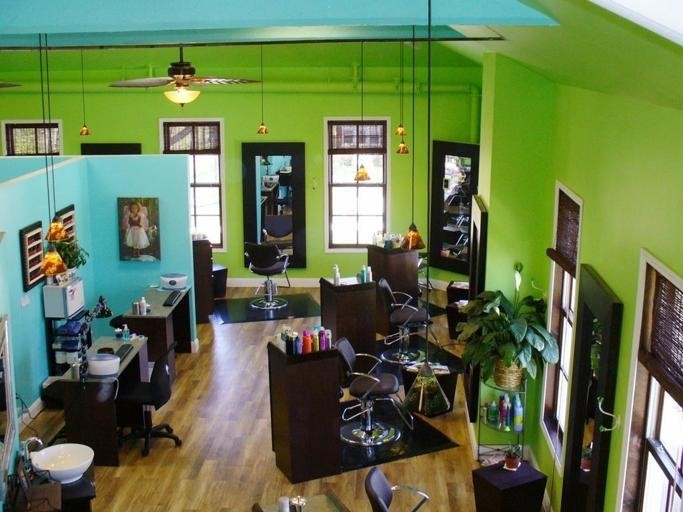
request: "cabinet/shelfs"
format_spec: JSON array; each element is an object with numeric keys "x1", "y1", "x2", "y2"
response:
[
  {"x1": 47, "y1": 311, "x2": 90, "y2": 377},
  {"x1": 269, "y1": 329, "x2": 342, "y2": 484},
  {"x1": 472, "y1": 459, "x2": 547, "y2": 512},
  {"x1": 321, "y1": 276, "x2": 381, "y2": 384},
  {"x1": 368, "y1": 245, "x2": 419, "y2": 337}
]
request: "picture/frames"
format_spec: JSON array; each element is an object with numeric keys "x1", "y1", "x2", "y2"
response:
[{"x1": 117, "y1": 197, "x2": 161, "y2": 262}]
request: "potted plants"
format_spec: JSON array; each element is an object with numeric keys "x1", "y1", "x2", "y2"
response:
[
  {"x1": 579, "y1": 448, "x2": 593, "y2": 470},
  {"x1": 503, "y1": 446, "x2": 521, "y2": 469},
  {"x1": 460, "y1": 273, "x2": 559, "y2": 388}
]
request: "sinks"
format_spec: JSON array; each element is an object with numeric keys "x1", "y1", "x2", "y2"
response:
[{"x1": 29, "y1": 443, "x2": 94, "y2": 485}]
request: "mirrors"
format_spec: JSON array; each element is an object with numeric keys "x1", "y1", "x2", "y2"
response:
[
  {"x1": 241, "y1": 141, "x2": 307, "y2": 271},
  {"x1": 427, "y1": 140, "x2": 480, "y2": 275},
  {"x1": 558, "y1": 263, "x2": 623, "y2": 512},
  {"x1": 462, "y1": 195, "x2": 487, "y2": 423},
  {"x1": 0, "y1": 315, "x2": 15, "y2": 500}
]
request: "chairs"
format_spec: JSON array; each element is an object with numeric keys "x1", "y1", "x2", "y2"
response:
[
  {"x1": 244, "y1": 244, "x2": 289, "y2": 311},
  {"x1": 451, "y1": 225, "x2": 471, "y2": 246},
  {"x1": 377, "y1": 278, "x2": 433, "y2": 363},
  {"x1": 367, "y1": 467, "x2": 429, "y2": 512},
  {"x1": 115, "y1": 343, "x2": 181, "y2": 457},
  {"x1": 334, "y1": 338, "x2": 414, "y2": 447},
  {"x1": 261, "y1": 214, "x2": 293, "y2": 249}
]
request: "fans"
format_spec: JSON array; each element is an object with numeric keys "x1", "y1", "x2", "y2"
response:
[
  {"x1": 109, "y1": 45, "x2": 262, "y2": 109},
  {"x1": 55, "y1": 242, "x2": 89, "y2": 280}
]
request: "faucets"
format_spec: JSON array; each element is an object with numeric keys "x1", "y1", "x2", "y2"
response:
[{"x1": 24, "y1": 436, "x2": 42, "y2": 474}]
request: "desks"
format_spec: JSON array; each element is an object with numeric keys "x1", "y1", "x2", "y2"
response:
[
  {"x1": 125, "y1": 286, "x2": 191, "y2": 373},
  {"x1": 61, "y1": 336, "x2": 148, "y2": 464}
]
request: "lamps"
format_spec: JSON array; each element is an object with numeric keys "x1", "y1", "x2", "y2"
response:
[
  {"x1": 396, "y1": 42, "x2": 409, "y2": 156},
  {"x1": 46, "y1": 34, "x2": 67, "y2": 243},
  {"x1": 408, "y1": 0, "x2": 451, "y2": 417},
  {"x1": 390, "y1": 42, "x2": 407, "y2": 136},
  {"x1": 40, "y1": 33, "x2": 67, "y2": 277},
  {"x1": 256, "y1": 45, "x2": 268, "y2": 135},
  {"x1": 78, "y1": 48, "x2": 91, "y2": 138},
  {"x1": 162, "y1": 83, "x2": 200, "y2": 109},
  {"x1": 354, "y1": 41, "x2": 367, "y2": 181},
  {"x1": 400, "y1": 26, "x2": 425, "y2": 250}
]
